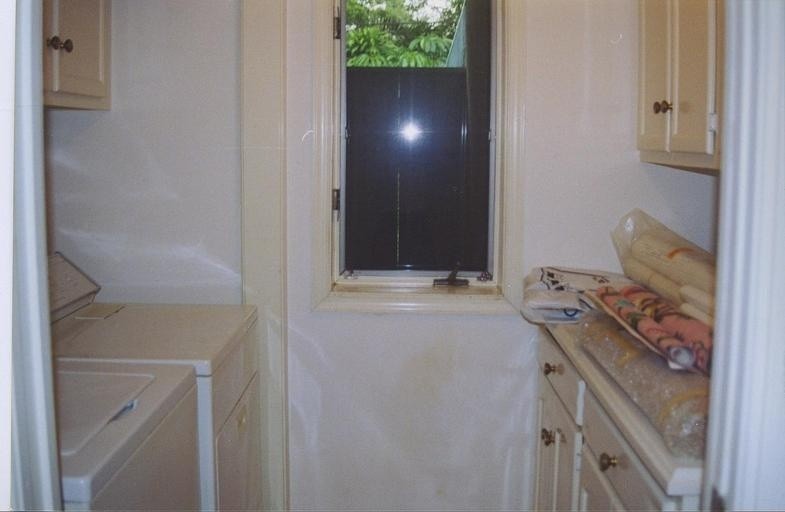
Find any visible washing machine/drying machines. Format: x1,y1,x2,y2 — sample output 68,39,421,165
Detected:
48,252,263,512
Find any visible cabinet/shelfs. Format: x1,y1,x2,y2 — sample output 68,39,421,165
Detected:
51,303,257,511
536,324,703,512
44,0,110,108
639,0,725,165
